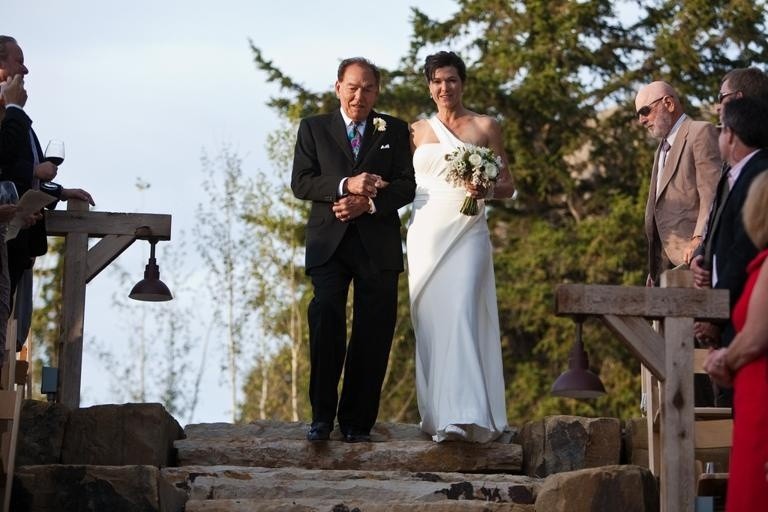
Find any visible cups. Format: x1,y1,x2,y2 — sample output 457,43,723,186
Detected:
703,462,722,475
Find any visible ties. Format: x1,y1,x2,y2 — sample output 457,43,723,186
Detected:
662,140,670,166
348,121,362,162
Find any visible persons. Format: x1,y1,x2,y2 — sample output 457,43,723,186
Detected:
689,98,768,408
375,51,515,443
702,168,767,511
715,66,767,120
635,80,723,286
291,55,417,442
0,35,96,376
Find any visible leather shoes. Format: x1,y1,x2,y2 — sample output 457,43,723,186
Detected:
346,434,370,442
308,422,332,440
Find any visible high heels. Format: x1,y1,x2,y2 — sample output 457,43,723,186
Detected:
445,424,468,441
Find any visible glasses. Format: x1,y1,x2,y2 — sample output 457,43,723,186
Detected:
717,91,736,104
636,95,674,120
715,123,725,132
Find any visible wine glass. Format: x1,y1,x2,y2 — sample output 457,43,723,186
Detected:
0,181,19,234
42,140,65,190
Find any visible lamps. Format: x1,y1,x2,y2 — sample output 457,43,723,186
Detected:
128,242,173,303
550,323,607,399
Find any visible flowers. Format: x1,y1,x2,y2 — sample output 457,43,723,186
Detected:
367,117,390,138
441,141,505,216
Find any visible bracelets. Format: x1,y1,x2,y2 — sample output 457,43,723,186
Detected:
485,189,495,200
